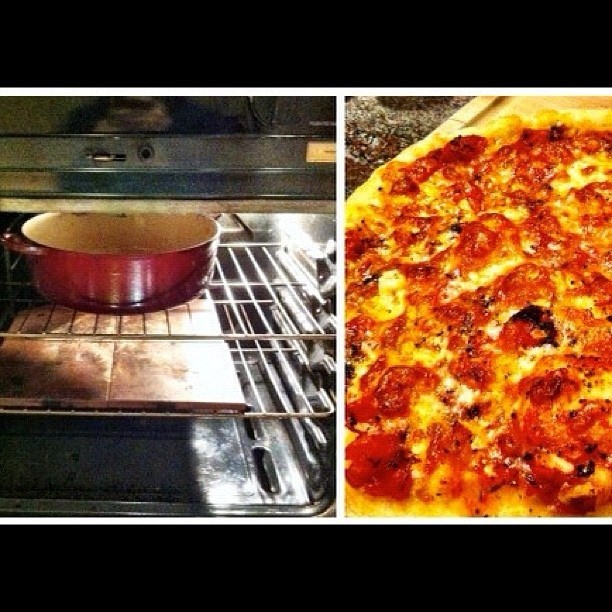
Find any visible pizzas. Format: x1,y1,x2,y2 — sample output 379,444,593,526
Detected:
342,106,611,519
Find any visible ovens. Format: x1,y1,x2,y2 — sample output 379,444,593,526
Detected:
1,97,336,517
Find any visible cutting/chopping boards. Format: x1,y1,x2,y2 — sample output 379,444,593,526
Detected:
0,304,250,412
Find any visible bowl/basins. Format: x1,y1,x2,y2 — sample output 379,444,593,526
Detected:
4,213,222,316
